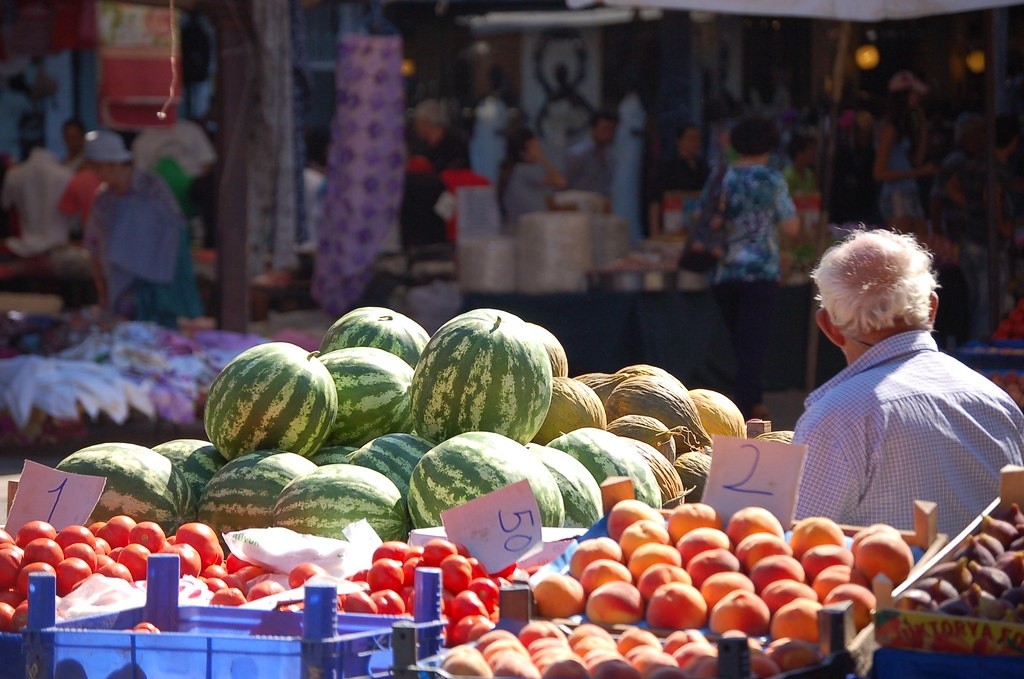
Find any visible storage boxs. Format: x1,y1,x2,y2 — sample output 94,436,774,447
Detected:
0,463,1024,679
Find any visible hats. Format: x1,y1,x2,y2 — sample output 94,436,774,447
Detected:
78,130,135,164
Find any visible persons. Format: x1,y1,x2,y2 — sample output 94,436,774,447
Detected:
790,232,1024,540
692,117,799,420
83,131,184,327
295,39,1024,349
0,118,216,318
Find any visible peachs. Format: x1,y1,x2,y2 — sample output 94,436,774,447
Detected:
445,496,913,678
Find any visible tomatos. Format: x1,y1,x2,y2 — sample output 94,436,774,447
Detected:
0,509,519,648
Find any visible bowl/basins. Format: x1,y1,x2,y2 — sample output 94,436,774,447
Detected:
602,265,677,293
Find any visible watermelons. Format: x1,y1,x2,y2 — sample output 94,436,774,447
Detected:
53,301,663,560
